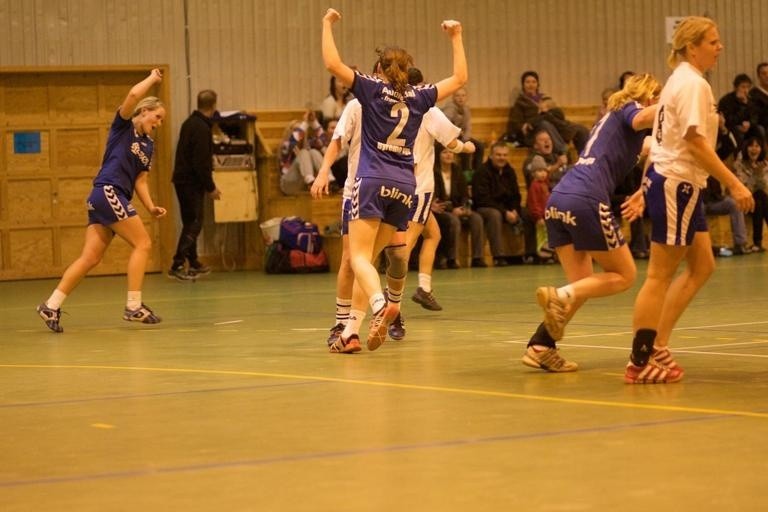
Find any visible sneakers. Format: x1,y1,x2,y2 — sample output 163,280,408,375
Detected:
650,344,685,374
168,267,192,283
306,180,341,194
411,286,442,312
623,353,684,387
388,310,406,340
367,303,398,351
519,345,579,373
35,302,64,333
188,265,212,279
326,323,346,346
122,301,162,324
535,285,573,342
328,333,362,354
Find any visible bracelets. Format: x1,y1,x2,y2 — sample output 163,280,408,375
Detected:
447,140,464,155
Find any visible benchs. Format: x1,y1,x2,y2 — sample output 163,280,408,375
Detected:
408,103,605,267
254,102,344,273
620,105,768,258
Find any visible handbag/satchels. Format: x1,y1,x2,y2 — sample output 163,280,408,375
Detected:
264,242,330,275
279,216,321,255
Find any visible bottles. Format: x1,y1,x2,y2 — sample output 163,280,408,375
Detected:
461,199,473,221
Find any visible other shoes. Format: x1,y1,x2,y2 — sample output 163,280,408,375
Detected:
748,245,761,253
471,257,489,268
498,258,508,266
522,255,543,265
541,258,554,264
631,248,651,258
446,259,461,269
733,245,747,253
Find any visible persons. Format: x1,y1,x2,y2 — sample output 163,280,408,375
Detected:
618,12,756,389
383,67,479,312
166,87,229,283
516,67,662,373
319,8,469,354
307,56,406,348
32,67,164,333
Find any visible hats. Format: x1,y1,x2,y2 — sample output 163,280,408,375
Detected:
530,155,548,173
